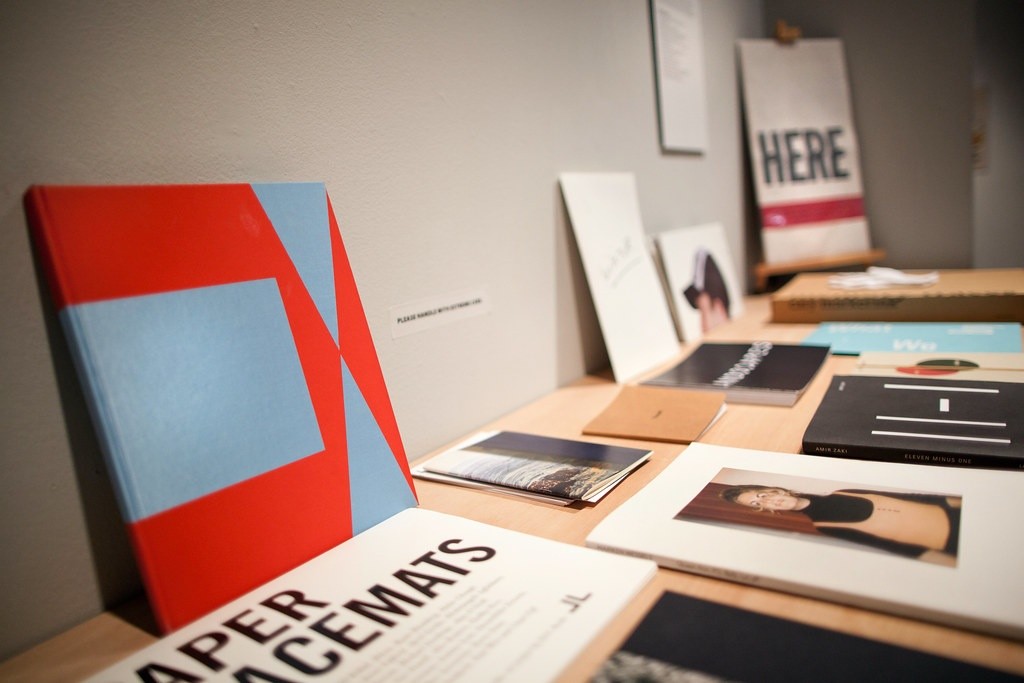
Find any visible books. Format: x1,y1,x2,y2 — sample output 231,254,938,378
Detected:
584,440,1023,632
81,501,1023,682
657,219,748,344
582,384,729,445
404,428,653,509
803,320,1023,373
801,374,1023,470
558,170,682,383
640,342,831,408
26,179,423,636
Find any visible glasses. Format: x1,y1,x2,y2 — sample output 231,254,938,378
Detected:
750,492,768,513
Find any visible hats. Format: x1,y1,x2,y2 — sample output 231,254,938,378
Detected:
683,250,731,318
